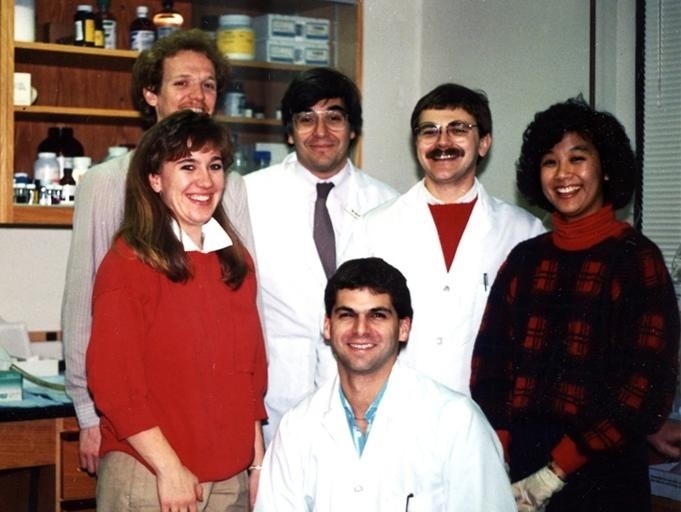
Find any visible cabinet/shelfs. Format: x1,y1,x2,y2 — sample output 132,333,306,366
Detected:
1,1,363,230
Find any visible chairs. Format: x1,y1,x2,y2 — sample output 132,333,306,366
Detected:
50,417,98,510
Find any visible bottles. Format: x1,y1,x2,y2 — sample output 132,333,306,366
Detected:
94,16,106,49
201,13,255,63
224,135,272,178
96,1,118,50
128,6,158,53
152,1,185,42
13,127,138,206
224,76,266,122
74,5,96,48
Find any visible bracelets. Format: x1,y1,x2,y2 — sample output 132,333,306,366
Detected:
546,463,570,486
245,462,267,472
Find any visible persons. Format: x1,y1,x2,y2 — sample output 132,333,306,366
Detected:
244,65,405,447
54,27,258,476
361,82,549,357
469,91,681,511
83,107,272,511
252,255,521,512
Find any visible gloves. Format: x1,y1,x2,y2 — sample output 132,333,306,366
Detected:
509,461,569,512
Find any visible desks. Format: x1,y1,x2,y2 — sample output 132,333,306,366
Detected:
0,348,104,512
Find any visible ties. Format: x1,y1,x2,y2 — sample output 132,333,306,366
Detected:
314,183,336,281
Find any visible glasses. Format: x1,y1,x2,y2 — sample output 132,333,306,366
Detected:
291,111,349,134
412,122,477,145
